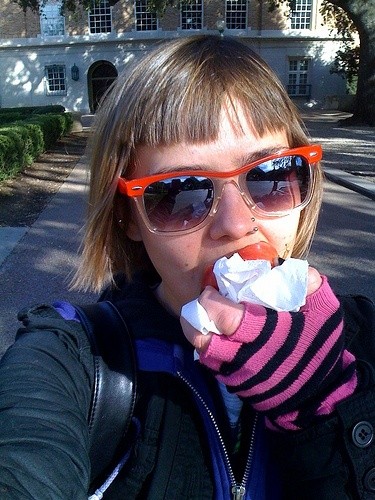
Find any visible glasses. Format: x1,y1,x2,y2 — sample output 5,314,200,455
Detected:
116,143,323,237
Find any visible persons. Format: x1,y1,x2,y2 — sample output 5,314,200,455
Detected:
147,183,183,232
1,34,374,500
